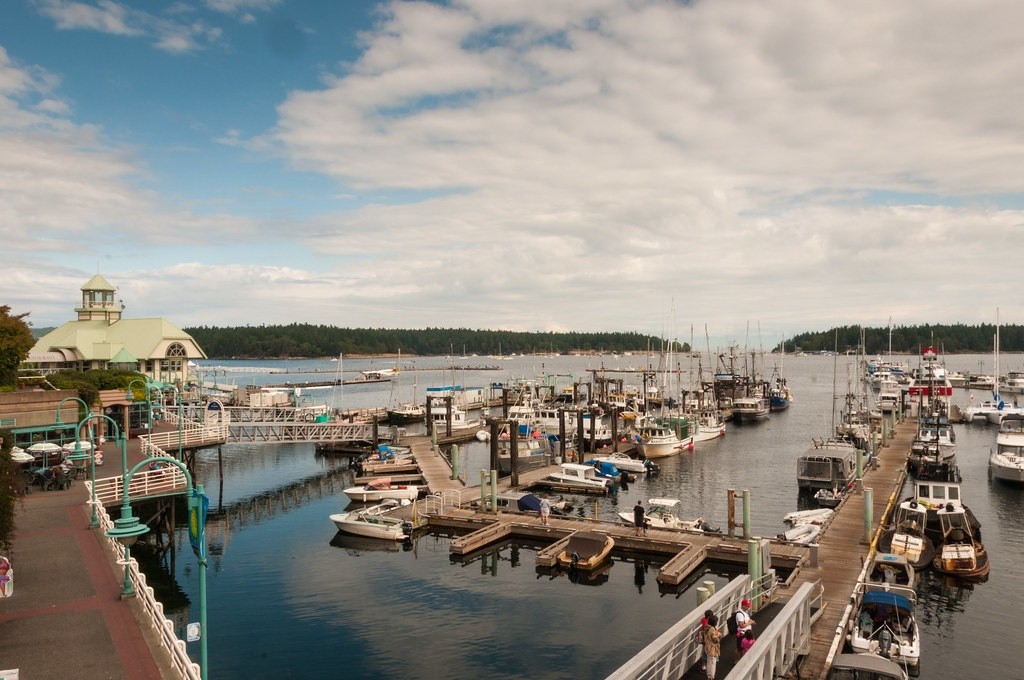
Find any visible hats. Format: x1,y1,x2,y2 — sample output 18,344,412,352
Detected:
741,599,752,607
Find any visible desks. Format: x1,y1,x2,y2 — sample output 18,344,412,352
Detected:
74,466,87,480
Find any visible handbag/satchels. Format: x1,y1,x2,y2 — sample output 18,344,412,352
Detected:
695,625,706,644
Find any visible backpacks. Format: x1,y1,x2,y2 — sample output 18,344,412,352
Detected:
727,611,745,635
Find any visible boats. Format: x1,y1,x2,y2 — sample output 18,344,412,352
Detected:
557,530,616,570
496,318,795,472
424,402,466,426
329,506,415,541
475,490,569,517
618,497,724,535
775,354,1024,680
584,451,662,483
343,476,420,502
387,404,426,424
537,462,616,495
355,446,414,468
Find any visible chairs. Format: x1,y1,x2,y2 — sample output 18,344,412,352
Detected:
22,463,92,493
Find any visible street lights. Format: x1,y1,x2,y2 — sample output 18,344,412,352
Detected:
184,373,218,422
152,384,183,463
52,396,99,525
65,413,136,595
124,379,152,459
103,455,209,679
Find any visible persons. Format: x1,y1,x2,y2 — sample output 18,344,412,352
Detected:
734,600,756,665
634,499,644,536
635,564,645,593
539,494,550,526
390,426,400,445
154,410,160,426
60,459,70,476
699,610,722,680
1013,396,1018,408
